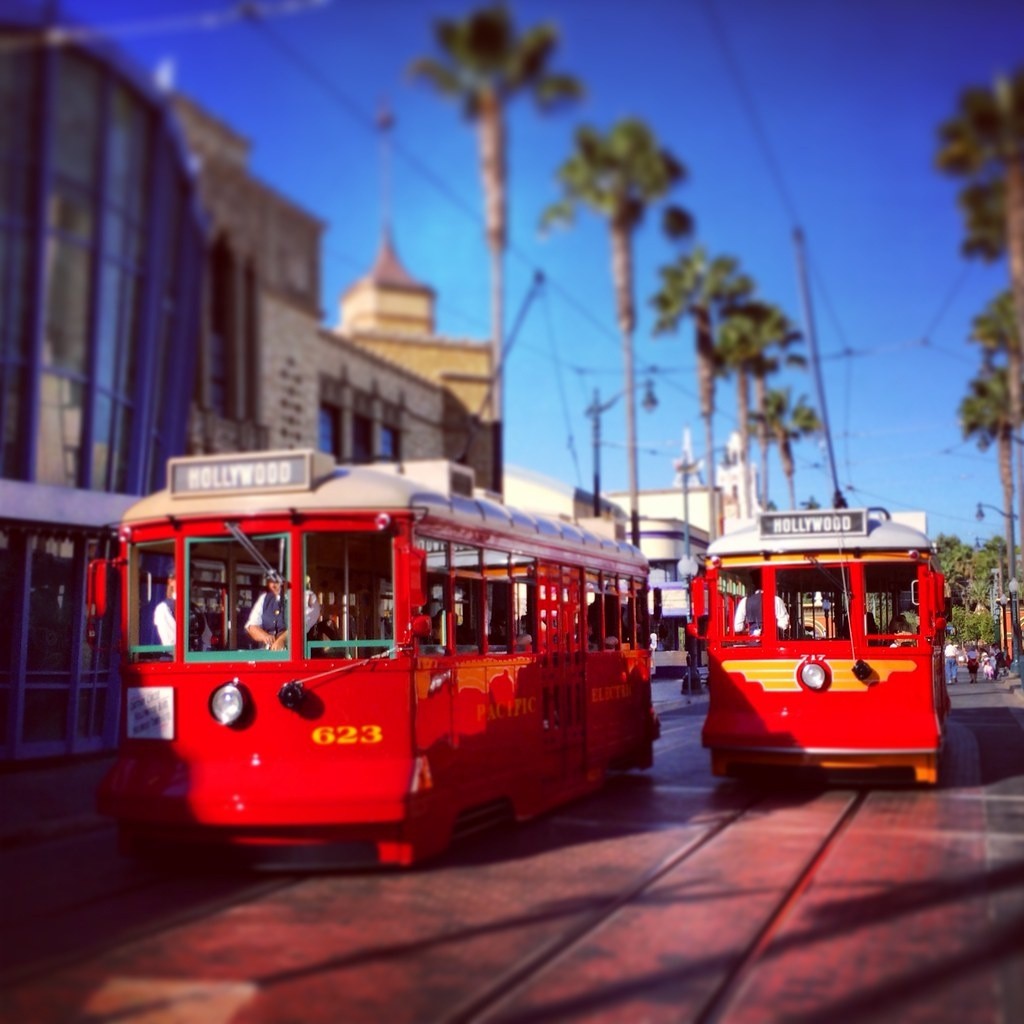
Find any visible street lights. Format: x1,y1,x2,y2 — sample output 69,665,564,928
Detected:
675,552,706,695
584,376,660,515
977,502,1017,576
1007,577,1022,674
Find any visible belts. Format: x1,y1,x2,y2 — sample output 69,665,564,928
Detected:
949,657,956,658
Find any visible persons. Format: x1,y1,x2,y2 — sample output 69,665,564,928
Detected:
944,640,1012,685
153,561,394,658
734,569,789,645
421,593,644,646
841,611,920,646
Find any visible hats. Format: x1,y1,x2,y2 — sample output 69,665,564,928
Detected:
167,561,195,577
262,566,279,586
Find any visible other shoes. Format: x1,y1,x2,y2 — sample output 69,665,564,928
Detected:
970,679,974,683
954,677,957,682
975,681,977,684
947,683,952,685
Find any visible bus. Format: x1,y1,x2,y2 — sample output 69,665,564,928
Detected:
88,451,660,870
687,508,957,793
